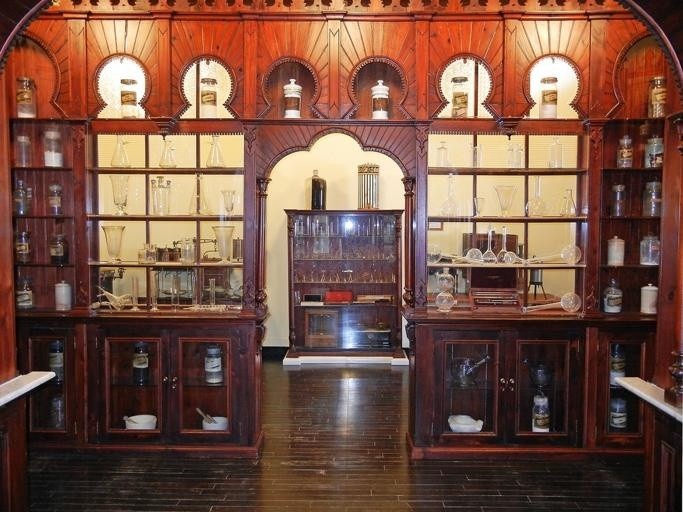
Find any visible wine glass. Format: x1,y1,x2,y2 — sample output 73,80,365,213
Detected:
450,358,479,389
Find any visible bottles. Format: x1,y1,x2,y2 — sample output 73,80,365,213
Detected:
603,277,659,315
199,77,219,118
48,341,64,381
437,172,577,218
614,134,663,168
14,131,72,314
282,78,303,118
437,134,562,169
532,360,546,384
647,76,668,119
609,344,627,387
295,216,398,283
450,76,469,119
606,232,661,265
311,170,325,211
539,76,559,118
133,344,149,385
204,347,222,384
119,77,140,120
46,395,65,431
370,79,390,119
429,223,582,267
611,393,630,431
531,395,550,433
101,133,234,312
607,178,662,218
428,268,581,314
16,77,37,119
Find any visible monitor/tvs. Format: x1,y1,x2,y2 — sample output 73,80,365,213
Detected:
468,265,519,292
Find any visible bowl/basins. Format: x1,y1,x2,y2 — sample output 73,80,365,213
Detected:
448,414,482,432
201,416,229,430
122,414,157,431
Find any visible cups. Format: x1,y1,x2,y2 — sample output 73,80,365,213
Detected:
391,296,395,305
